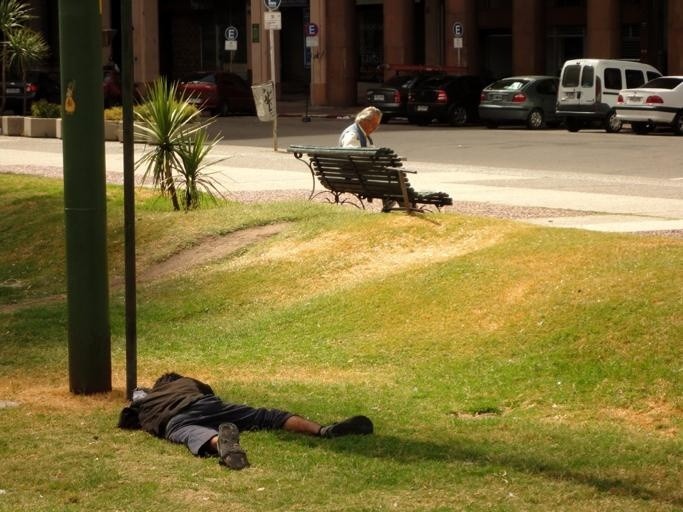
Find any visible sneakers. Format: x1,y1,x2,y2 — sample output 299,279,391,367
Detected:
217,422,250,470
319,416,373,438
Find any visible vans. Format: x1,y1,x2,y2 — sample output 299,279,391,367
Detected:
554,59,663,132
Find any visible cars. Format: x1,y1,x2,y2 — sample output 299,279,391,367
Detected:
478,75,560,129
102,65,121,102
174,72,252,117
1,70,59,113
366,71,446,120
615,76,682,136
404,73,504,127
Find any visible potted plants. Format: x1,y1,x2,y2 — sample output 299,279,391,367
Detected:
22,99,203,146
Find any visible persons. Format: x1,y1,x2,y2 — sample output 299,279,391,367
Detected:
338,106,416,212
119,373,372,470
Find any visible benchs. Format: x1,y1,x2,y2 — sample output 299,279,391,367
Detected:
285,143,453,216
